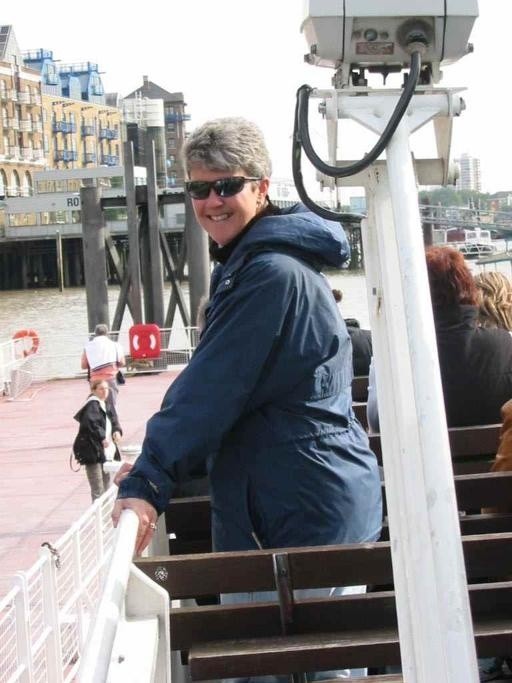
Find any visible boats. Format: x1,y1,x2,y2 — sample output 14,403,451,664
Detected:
473,238,512,266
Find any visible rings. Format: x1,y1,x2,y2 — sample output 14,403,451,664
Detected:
141,514,149,525
154,526,157,529
149,522,156,528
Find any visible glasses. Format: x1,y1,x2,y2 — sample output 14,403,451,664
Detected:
184,175,263,200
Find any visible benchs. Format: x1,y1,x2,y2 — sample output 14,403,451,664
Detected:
367,423,512,541
170,471,511,606
134,532,512,682
351,376,370,431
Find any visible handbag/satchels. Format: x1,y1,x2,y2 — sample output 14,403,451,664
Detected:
74,433,102,465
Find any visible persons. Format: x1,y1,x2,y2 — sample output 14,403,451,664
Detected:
72,378,123,499
367,242,512,434
336,198,341,208
481,399,512,586
112,116,386,682
472,270,512,331
79,322,126,404
344,315,372,375
331,287,342,304
